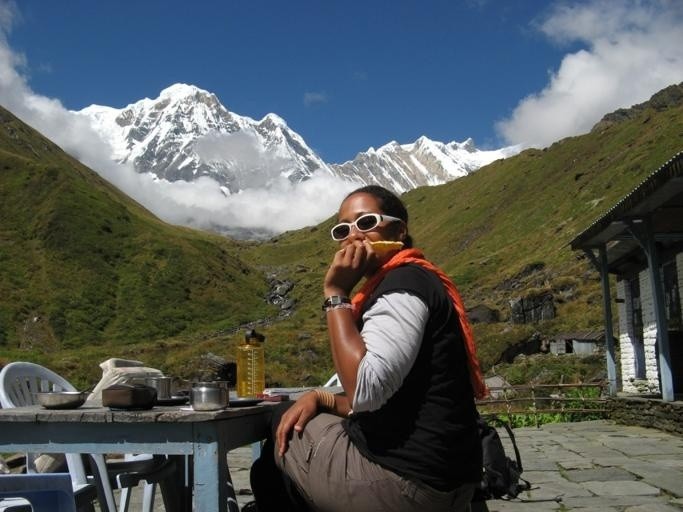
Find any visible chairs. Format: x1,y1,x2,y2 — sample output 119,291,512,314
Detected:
0,360,177,512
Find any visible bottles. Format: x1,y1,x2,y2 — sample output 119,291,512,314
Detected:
236,328,265,399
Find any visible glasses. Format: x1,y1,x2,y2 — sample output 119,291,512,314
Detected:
330,213,400,241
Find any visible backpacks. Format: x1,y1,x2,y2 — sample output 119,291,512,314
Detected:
479,417,530,497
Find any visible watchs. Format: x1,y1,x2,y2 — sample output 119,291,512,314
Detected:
320,294,351,311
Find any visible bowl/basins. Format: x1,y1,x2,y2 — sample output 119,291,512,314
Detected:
30,390,90,411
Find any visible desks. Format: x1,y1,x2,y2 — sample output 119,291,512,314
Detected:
0,386,346,512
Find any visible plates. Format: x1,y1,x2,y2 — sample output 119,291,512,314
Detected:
229,398,266,407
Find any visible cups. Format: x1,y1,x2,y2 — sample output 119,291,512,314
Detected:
145,375,172,401
189,380,231,412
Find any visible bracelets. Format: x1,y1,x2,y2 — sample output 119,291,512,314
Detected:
312,388,335,412
325,302,356,312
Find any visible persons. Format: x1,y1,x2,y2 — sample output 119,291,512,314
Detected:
249,186,489,511
